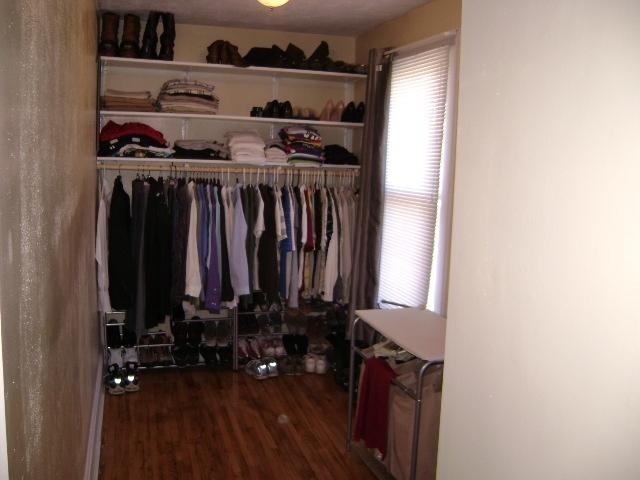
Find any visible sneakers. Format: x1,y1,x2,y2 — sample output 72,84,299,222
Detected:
171,287,350,383
107,348,123,370
139,333,155,367
154,330,172,366
103,364,124,396
107,318,121,347
123,360,141,393
122,317,138,348
124,347,138,370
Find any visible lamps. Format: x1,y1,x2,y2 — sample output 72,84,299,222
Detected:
256,1,289,9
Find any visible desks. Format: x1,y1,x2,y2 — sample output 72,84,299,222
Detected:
344,308,444,480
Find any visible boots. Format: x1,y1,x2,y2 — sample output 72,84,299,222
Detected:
119,12,142,58
157,10,177,61
140,7,159,61
98,11,122,59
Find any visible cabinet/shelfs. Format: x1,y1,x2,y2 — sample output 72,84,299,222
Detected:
95,55,371,370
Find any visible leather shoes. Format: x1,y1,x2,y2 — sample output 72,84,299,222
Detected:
204,38,252,69
261,95,366,124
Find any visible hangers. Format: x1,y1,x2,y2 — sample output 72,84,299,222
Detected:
96,162,358,199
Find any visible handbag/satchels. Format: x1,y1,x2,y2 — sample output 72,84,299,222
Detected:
352,347,444,480
